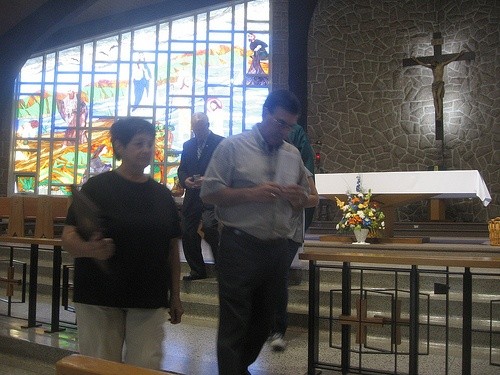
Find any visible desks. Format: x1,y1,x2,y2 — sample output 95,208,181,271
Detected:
315,169,492,221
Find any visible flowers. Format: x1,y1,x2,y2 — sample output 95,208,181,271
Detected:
333,175,386,232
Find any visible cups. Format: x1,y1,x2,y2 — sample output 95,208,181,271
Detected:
193,173,201,187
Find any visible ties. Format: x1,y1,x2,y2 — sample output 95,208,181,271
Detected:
197,143,203,159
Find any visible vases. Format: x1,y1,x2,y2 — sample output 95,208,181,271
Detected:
352,229,370,245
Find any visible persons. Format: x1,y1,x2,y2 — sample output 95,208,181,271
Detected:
198,89,320,375
265,124,320,349
62,116,184,371
177,113,225,281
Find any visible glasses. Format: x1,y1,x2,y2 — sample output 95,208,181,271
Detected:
273,117,296,134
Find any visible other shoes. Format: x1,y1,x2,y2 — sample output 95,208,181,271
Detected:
270,332,287,351
183,274,207,280
